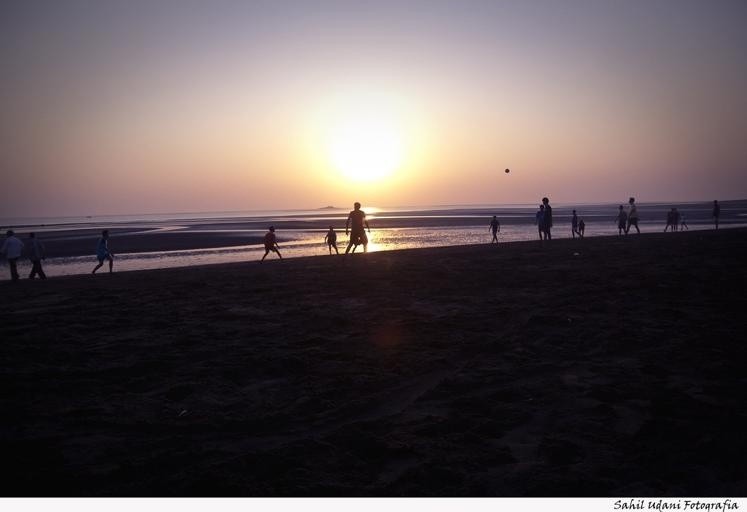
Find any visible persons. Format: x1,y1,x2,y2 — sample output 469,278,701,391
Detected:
324,225,339,255
344,202,371,255
0,230,25,281
487,195,722,243
91,227,115,274
258,224,285,264
23,231,48,279
352,236,368,255
